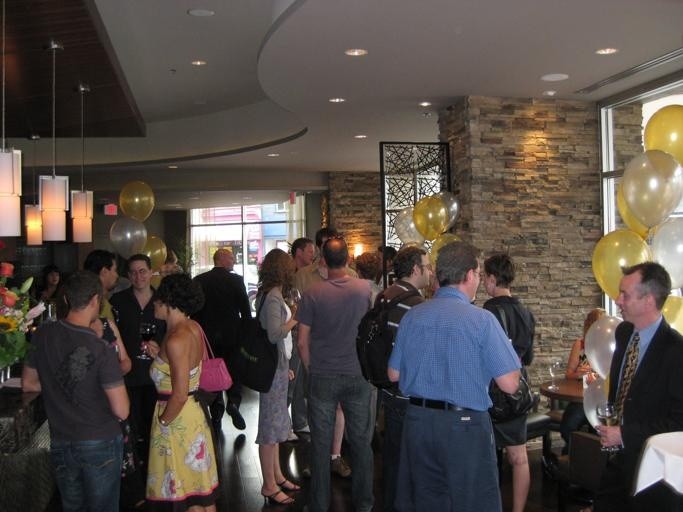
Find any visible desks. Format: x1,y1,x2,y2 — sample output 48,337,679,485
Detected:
540,379,583,412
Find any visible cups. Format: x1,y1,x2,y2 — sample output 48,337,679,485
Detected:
287,287,301,304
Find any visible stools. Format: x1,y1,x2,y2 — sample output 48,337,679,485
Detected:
496,412,551,492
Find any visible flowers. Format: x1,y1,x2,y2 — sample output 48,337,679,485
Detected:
0,263,46,368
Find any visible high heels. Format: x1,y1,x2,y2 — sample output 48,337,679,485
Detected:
277,478,302,492
261,488,294,507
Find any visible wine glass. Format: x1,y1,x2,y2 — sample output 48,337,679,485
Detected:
594,401,623,453
135,320,160,361
546,358,561,392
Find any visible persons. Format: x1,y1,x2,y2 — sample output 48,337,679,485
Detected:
256,228,381,511
387,241,523,511
594,262,683,512
140,273,219,511
484,254,535,511
560,307,606,457
23,249,132,511
109,255,167,511
191,249,252,435
371,246,430,510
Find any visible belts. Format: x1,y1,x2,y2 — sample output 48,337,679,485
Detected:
409,396,473,412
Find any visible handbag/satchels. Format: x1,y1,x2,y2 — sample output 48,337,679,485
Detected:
201,358,232,392
231,317,278,392
490,374,534,422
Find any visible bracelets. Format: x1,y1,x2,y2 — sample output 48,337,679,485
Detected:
159,417,168,426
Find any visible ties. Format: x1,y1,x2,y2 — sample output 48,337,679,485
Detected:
615,332,640,425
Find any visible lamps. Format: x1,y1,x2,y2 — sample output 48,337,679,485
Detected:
38,43,69,242
352,244,364,261
103,203,118,216
72,85,93,243
0,1,22,237
24,136,42,245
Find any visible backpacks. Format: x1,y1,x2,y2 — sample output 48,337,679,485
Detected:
356,291,420,389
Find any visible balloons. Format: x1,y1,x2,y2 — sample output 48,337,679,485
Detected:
393,190,463,272
109,180,167,274
582,104,683,433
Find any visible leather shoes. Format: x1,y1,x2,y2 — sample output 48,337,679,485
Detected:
226,401,246,429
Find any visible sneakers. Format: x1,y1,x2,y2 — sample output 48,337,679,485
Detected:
286,429,297,441
330,456,353,478
